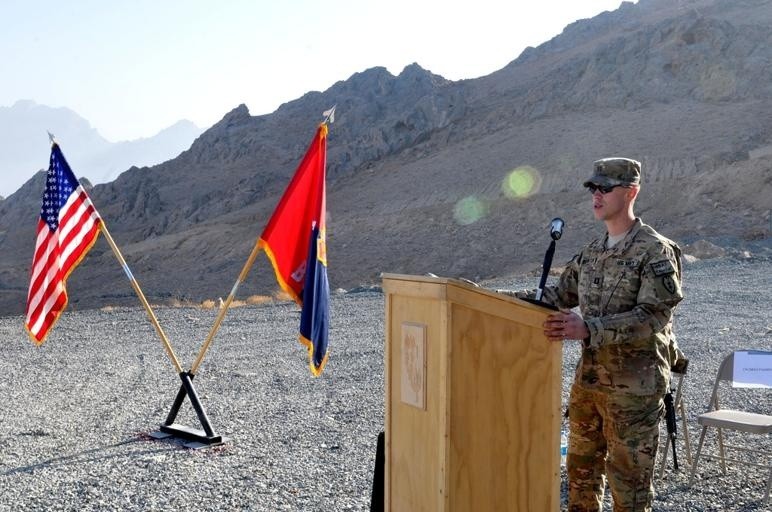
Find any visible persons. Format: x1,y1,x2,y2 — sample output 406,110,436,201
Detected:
490,157,684,510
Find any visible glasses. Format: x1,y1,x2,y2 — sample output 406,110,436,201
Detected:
589,183,621,194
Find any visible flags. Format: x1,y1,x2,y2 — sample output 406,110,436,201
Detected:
257,121,330,380
22,142,104,347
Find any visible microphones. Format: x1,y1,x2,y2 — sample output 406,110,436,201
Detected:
550,218,564,243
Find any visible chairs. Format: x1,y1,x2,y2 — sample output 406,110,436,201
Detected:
692,347,771,489
621,353,695,479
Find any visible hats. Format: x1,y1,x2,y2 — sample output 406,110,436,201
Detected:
583,157,641,188
